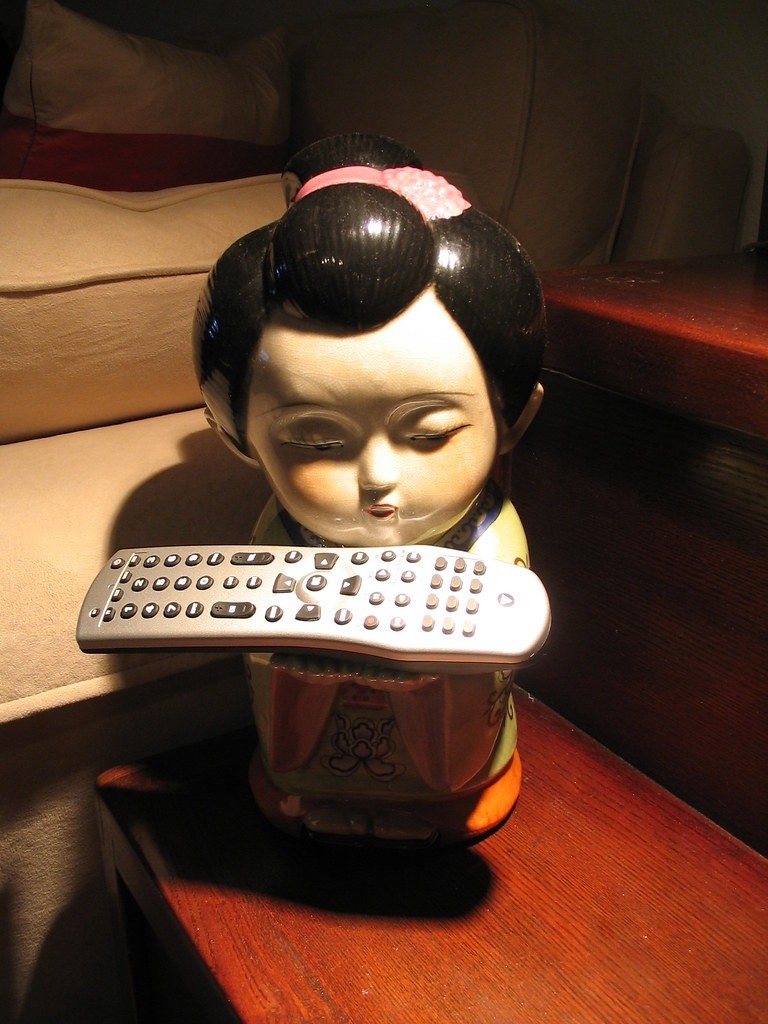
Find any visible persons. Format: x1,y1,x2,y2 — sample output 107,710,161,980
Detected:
193,133,549,843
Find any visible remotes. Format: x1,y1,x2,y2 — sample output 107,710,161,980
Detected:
75,545,553,680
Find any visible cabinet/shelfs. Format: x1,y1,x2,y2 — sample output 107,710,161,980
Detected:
512,252,768,851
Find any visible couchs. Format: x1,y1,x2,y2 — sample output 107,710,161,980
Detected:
0,0,752,752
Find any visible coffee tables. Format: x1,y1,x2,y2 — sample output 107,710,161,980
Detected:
94,685,768,1024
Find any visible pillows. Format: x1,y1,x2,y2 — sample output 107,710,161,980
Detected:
3,0,294,155
